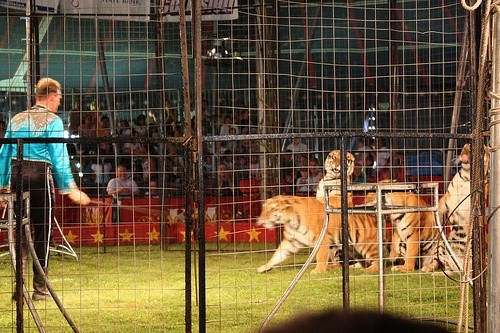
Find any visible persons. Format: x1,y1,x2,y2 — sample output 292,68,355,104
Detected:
69,97,405,198
0,77,90,303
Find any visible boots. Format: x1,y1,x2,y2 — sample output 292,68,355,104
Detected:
32,267,54,301
